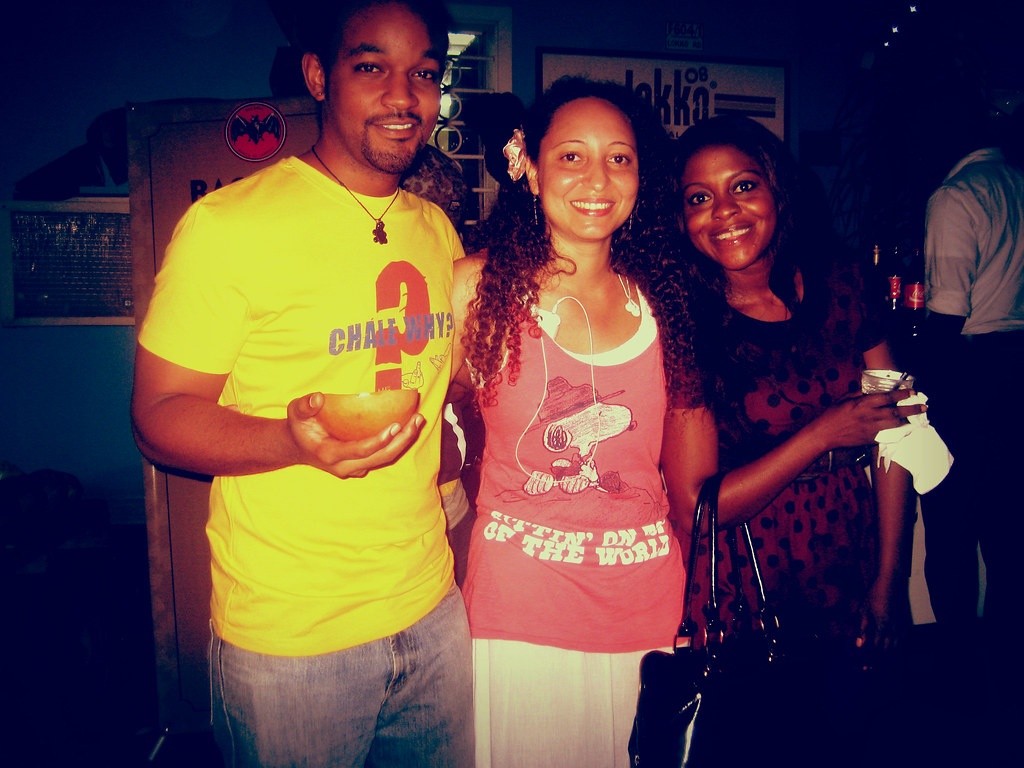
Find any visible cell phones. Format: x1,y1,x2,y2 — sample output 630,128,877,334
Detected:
530,306,560,341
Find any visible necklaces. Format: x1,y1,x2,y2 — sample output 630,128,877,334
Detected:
312,145,399,243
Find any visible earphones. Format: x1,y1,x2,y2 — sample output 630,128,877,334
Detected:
632,303,641,317
625,299,635,312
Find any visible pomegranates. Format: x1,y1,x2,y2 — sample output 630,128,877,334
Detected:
312,388,417,441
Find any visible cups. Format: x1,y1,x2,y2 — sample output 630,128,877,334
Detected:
861,369,915,393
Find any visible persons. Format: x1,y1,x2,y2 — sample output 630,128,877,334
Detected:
644,115,907,650
908,92,1024,660
440,77,688,768
130,0,475,768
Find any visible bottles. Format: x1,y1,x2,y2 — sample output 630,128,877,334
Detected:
875,246,925,345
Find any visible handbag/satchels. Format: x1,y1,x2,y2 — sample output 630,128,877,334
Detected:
628,471,814,768
